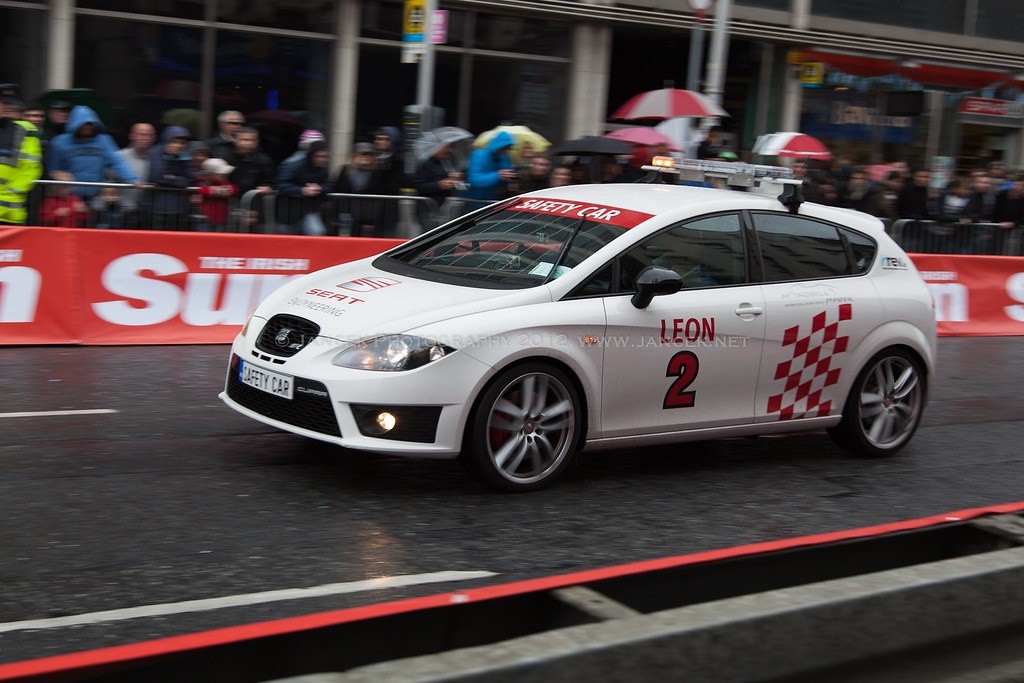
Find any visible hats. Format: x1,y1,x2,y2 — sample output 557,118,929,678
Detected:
0,83,29,105
297,129,327,145
367,127,403,146
199,157,237,175
354,142,377,154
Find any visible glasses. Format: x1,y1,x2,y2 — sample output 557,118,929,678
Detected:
219,121,247,127
375,136,392,142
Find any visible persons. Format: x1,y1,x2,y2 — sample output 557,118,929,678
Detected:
465,132,520,212
196,159,239,236
113,122,156,227
27,87,94,225
0,84,43,225
209,112,246,157
648,120,1024,239
405,142,465,232
520,153,555,194
187,139,210,167
335,126,403,237
549,165,571,188
274,140,330,236
47,106,144,226
225,130,268,199
144,126,199,229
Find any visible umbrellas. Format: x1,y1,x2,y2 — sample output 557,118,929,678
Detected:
611,87,732,119
413,127,473,190
549,136,633,155
753,132,832,161
604,128,684,152
474,124,551,156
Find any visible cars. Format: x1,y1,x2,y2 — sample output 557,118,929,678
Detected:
217,183,937,492
